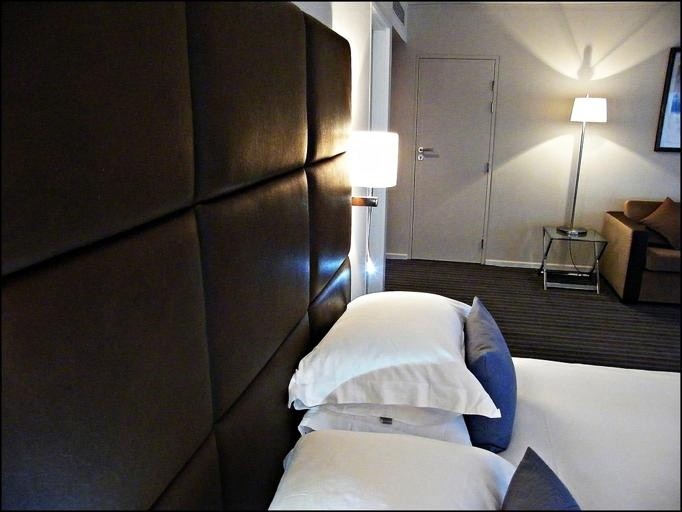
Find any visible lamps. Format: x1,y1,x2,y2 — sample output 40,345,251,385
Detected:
556,97,609,237
352,129,401,296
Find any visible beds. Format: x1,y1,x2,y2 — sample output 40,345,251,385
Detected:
473,357,680,512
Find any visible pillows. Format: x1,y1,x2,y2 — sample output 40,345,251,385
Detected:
298,409,472,446
638,197,681,250
463,296,518,452
268,430,516,511
502,446,582,511
288,290,502,424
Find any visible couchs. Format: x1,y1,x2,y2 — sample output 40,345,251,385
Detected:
596,200,680,307
